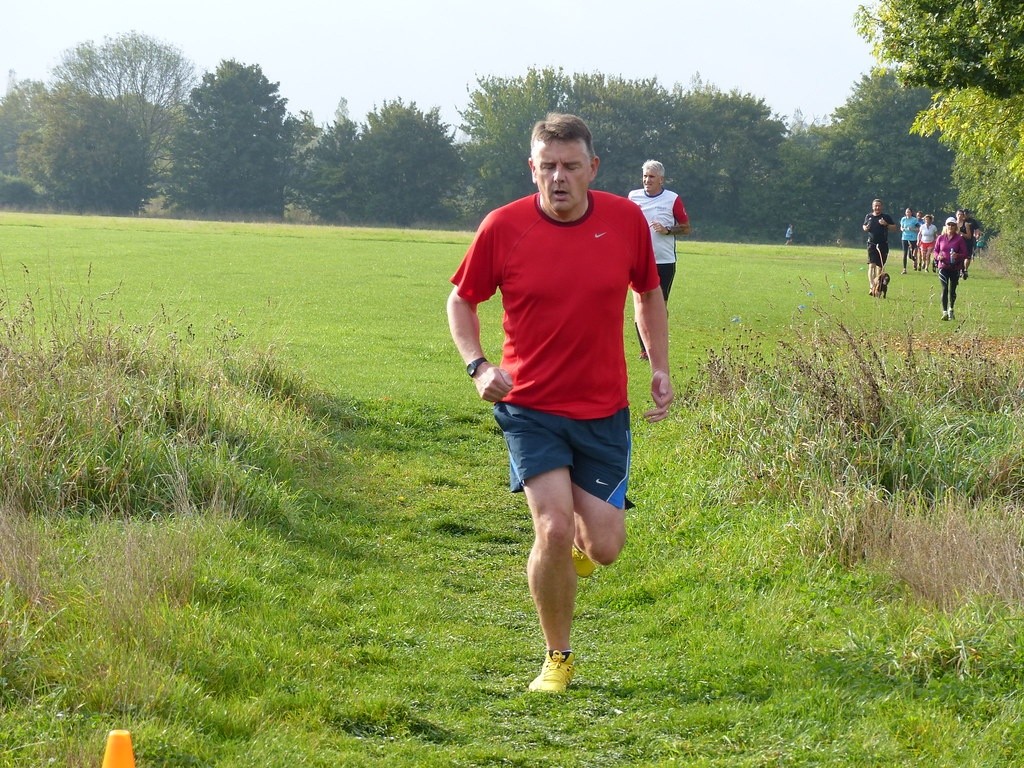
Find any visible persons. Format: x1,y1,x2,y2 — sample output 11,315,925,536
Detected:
862,199,897,297
446,112,673,693
900,208,919,274
909,211,937,273
785,224,794,245
934,217,967,321
628,159,691,360
956,209,989,280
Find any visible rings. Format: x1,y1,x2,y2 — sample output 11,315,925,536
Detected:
666,409,670,413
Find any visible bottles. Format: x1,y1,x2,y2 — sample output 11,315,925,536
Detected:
868,217,872,229
950,248,954,263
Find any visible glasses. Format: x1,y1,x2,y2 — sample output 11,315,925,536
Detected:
947,223,956,226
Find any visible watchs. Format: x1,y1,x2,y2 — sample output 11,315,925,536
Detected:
466,358,488,378
665,226,671,235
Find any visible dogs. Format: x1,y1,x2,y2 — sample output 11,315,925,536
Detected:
873,273,890,299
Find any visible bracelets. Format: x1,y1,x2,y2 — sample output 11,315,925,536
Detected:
884,222,889,227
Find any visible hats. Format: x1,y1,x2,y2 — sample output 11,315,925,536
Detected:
945,217,957,226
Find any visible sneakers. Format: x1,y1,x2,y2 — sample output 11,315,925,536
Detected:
572,544,595,579
529,651,575,694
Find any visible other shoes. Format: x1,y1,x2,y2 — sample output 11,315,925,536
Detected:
941,311,948,321
960,272,962,277
921,263,924,268
964,271,968,277
913,261,917,271
901,271,906,274
949,308,955,321
925,268,929,273
869,288,873,296
919,264,921,271
